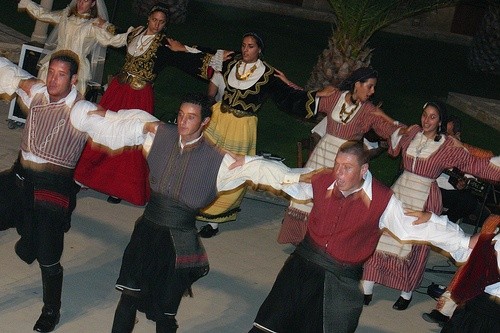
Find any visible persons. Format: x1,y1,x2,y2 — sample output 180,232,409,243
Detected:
88,93,333,333
0,57,45,97
220,139,497,333
165,31,341,239
92,4,234,204
272,67,418,254
0,49,164,332
18,0,133,98
403,207,500,333
434,117,496,224
362,101,500,311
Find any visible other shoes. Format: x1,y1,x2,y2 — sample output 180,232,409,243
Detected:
392,296,412,310
363,295,372,305
423,310,449,327
107,195,121,204
198,224,218,238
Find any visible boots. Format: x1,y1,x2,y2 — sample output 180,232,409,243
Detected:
156,320,178,333
33,266,63,333
111,293,139,333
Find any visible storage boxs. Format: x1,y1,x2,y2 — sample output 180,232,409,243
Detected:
8,41,58,124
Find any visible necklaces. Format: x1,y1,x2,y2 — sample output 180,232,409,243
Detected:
235,61,256,80
340,104,360,123
140,36,156,51
416,131,437,157
